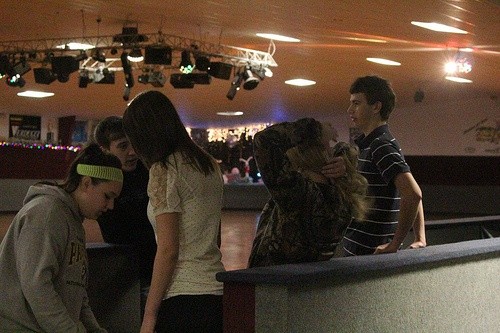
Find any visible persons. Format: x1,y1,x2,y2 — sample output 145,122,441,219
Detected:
342,76,427,258
122,90,226,333
0,138,124,333
94,116,157,323
246,117,369,268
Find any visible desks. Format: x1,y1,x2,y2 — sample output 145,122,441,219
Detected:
409,215,500,239
222,183,270,209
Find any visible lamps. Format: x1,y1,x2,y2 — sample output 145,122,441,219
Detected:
0,18,278,101
445,48,474,84
